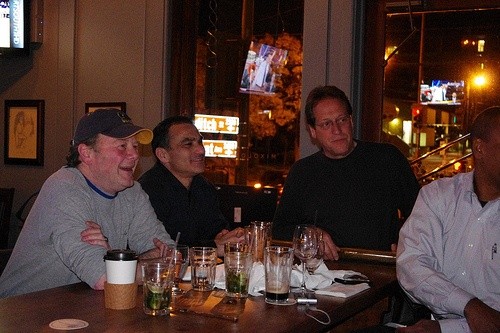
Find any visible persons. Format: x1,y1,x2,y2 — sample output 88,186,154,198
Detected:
273,86,422,260
396,106,500,333
137,115,251,256
0,107,176,300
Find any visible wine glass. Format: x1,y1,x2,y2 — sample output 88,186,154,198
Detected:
161,243,190,297
292,224,320,300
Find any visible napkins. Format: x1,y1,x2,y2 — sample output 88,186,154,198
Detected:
315,282,370,298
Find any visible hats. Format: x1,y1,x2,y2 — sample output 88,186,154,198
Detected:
74,108,154,145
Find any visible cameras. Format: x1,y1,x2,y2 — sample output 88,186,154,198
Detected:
294,292,317,305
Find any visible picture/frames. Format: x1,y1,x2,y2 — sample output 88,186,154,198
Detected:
3,98,45,167
85,102,126,114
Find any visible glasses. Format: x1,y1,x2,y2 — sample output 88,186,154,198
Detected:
314,113,349,131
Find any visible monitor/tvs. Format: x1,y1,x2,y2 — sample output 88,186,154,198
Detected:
0,0,30,55
237,38,289,96
421,78,465,106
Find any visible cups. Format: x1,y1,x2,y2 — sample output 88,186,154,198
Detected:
223,242,253,298
249,220,273,265
141,257,176,317
305,240,325,276
263,246,294,303
102,248,139,285
189,246,217,292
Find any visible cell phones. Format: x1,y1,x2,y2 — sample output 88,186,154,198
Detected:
334,277,371,284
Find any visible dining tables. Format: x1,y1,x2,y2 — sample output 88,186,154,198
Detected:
0,240,399,333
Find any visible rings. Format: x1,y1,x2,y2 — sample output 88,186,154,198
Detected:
103,235,108,242
